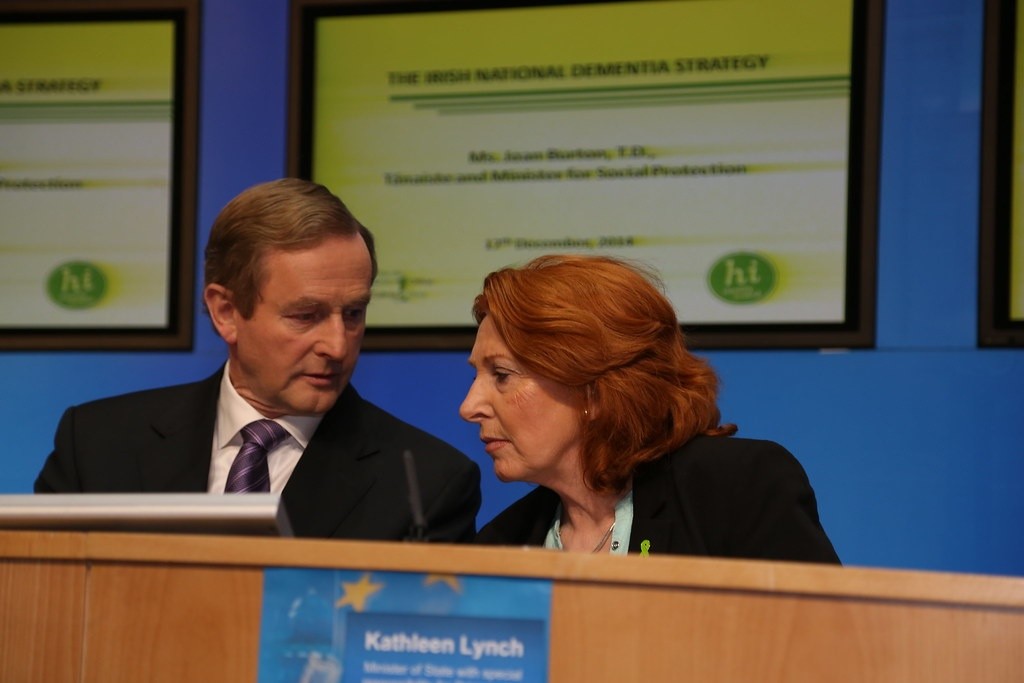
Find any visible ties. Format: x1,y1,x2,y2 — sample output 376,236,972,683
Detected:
224,419,292,493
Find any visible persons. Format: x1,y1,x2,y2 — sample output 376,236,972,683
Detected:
32,177,480,546
460,254,843,566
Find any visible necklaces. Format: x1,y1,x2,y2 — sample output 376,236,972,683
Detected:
592,522,616,553
